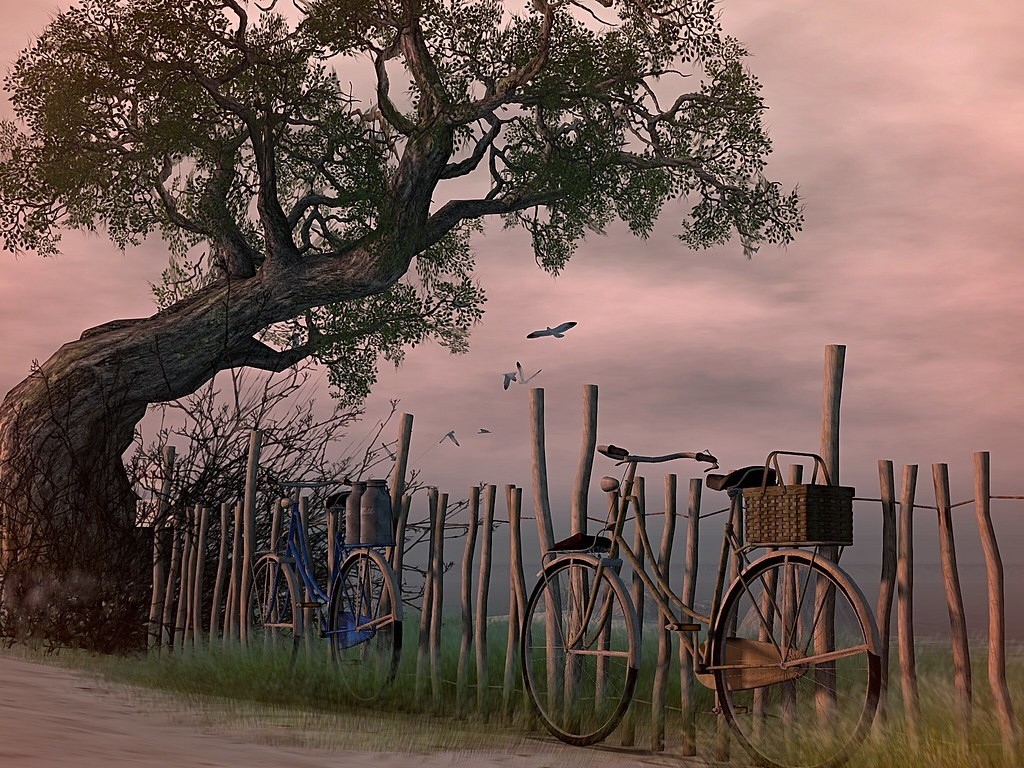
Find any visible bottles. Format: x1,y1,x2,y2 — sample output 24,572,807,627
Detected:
360,479,392,544
345,480,366,544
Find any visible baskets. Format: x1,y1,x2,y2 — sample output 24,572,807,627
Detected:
742,451,856,546
